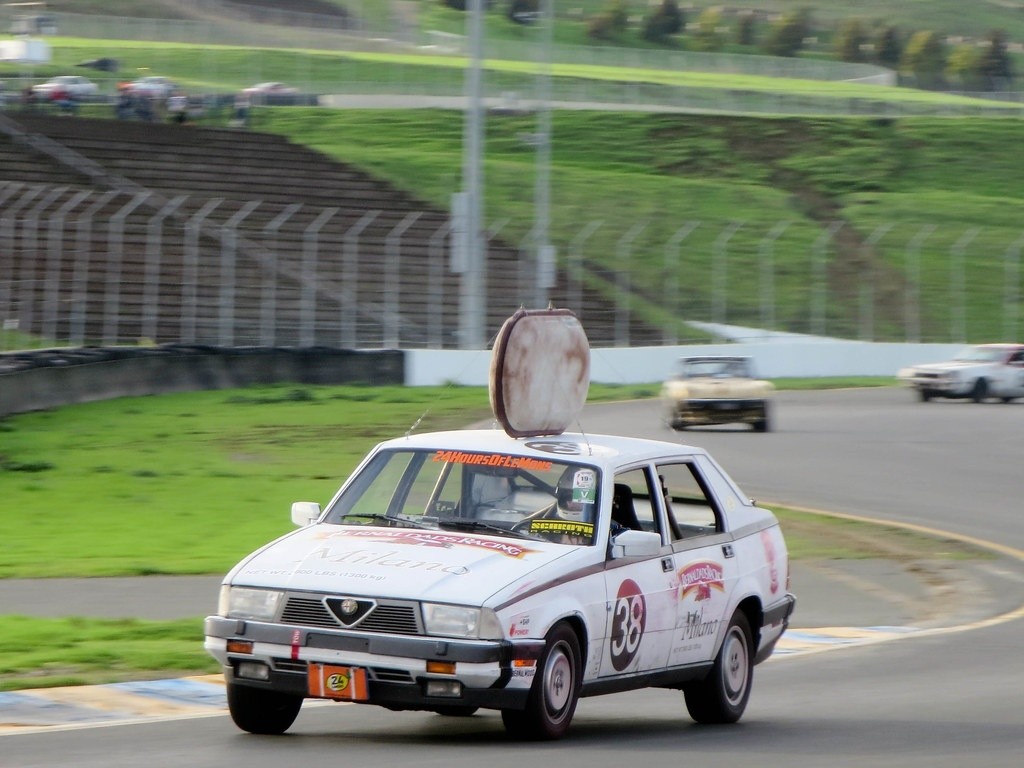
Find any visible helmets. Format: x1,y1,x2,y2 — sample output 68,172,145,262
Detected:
556,465,599,523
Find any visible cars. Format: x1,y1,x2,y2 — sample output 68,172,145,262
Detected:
28,75,98,103
203,430,796,743
240,81,321,108
898,344,1024,404
121,74,192,106
660,354,776,431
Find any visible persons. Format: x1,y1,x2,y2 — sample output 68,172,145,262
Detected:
517,467,632,544
